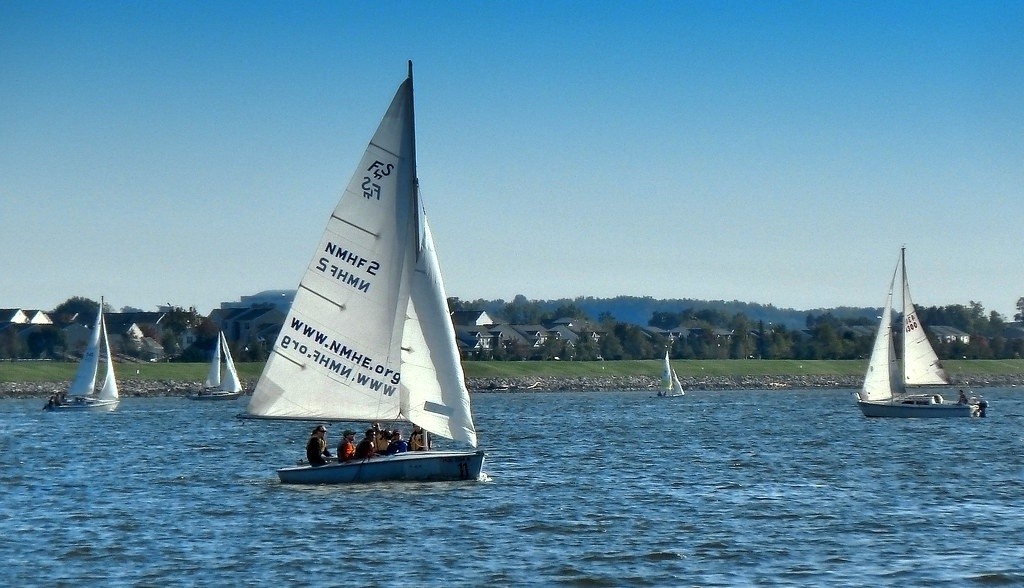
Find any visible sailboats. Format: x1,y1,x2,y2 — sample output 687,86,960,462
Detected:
651,350,686,399
244,59,488,487
186,330,245,401
850,245,990,418
42,294,120,414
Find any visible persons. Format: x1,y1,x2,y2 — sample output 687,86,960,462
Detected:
307,425,333,467
657,390,667,396
49,394,66,406
953,389,968,406
336,430,356,463
354,422,407,458
198,390,203,396
407,423,432,451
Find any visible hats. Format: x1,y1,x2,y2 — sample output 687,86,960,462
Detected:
343,430,356,436
392,429,401,435
313,425,326,435
